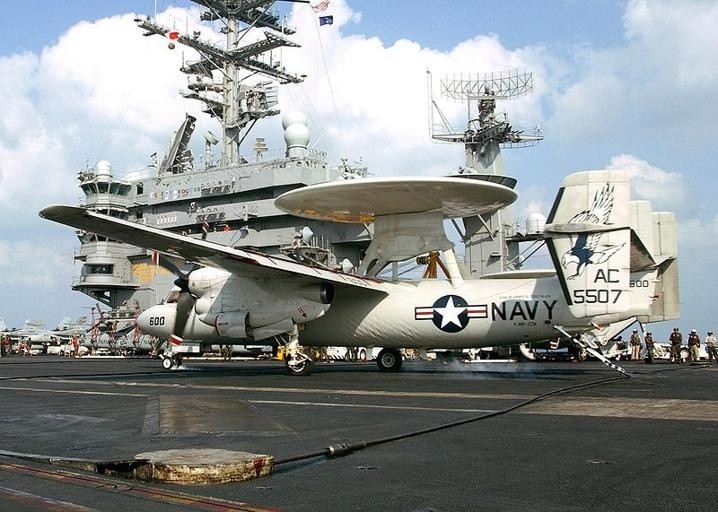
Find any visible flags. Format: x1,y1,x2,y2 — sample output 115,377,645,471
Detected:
319,15,334,26
312,0,331,13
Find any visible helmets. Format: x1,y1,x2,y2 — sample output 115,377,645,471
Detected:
647,331,652,335
707,329,713,335
674,328,679,332
633,329,637,333
691,329,697,334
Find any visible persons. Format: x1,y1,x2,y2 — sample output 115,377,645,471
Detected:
630,329,640,361
686,329,701,360
645,331,656,361
669,328,683,364
705,330,718,363
